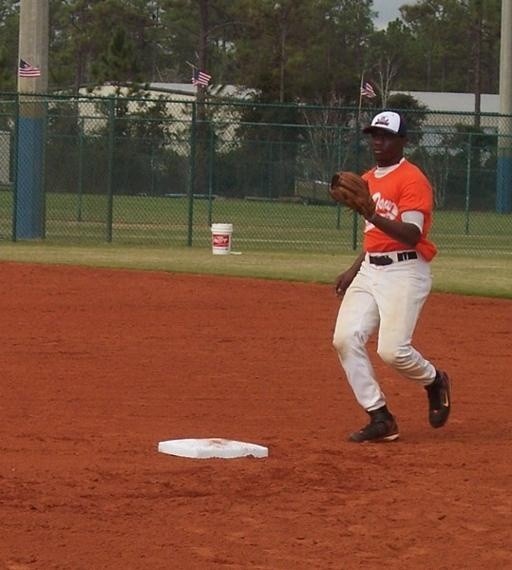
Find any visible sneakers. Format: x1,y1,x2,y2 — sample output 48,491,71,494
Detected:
424,370,450,428
350,416,399,442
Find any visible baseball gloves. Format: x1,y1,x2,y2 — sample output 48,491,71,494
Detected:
329,172,375,217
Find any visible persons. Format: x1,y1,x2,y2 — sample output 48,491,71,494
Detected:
330,112,449,443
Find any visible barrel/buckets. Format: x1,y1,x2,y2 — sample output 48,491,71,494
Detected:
210,223,234,256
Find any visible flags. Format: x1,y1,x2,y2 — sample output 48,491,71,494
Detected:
19,56,41,77
193,67,212,85
361,81,376,98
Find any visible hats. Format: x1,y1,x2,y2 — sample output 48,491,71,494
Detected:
363,109,406,137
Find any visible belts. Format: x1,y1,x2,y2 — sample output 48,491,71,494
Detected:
369,251,417,265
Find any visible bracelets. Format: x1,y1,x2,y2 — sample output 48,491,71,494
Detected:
369,212,378,220
